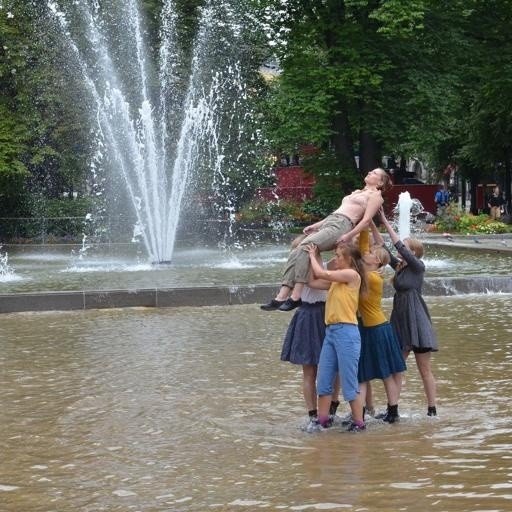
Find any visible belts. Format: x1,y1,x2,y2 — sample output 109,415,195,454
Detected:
331,212,355,230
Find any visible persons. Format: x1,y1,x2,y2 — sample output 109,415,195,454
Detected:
432,184,511,221
279,204,441,433
258,166,395,313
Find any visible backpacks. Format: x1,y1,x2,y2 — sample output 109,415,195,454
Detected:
434,191,444,203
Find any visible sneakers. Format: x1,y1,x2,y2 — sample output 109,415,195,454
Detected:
260,298,286,312
300,415,399,434
279,298,303,311
